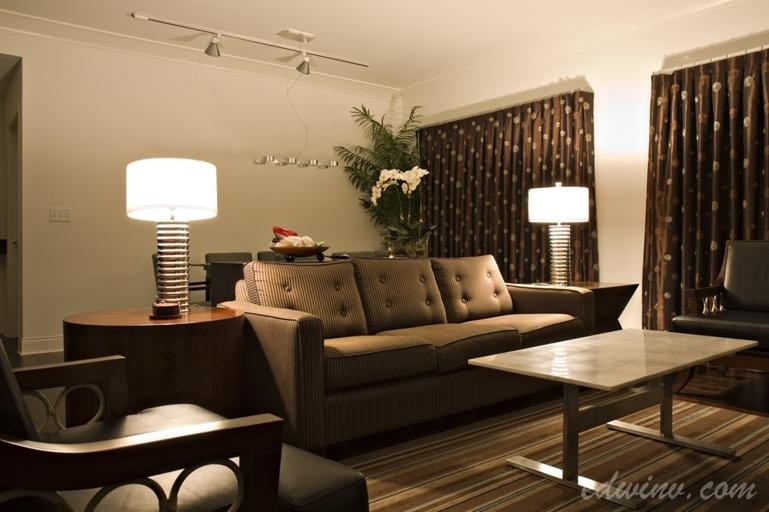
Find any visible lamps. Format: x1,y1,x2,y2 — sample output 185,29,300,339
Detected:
125,157,218,313
526,186,589,284
206,33,222,57
294,52,316,74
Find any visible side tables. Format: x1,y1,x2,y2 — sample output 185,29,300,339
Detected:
542,278,639,333
62,305,245,423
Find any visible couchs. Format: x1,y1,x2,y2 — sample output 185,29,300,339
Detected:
215,257,598,453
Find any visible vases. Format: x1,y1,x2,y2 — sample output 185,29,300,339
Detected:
385,224,434,258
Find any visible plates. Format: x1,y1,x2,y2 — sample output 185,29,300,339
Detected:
268,244,329,258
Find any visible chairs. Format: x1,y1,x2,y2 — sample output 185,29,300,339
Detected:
332,251,373,259
152,252,207,305
0,337,368,511
374,250,389,256
257,252,317,261
671,240,768,391
205,252,253,302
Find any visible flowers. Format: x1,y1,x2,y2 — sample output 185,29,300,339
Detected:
336,104,430,229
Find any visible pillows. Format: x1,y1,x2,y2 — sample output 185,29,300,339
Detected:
243,254,512,337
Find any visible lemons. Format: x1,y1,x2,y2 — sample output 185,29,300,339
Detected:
275,240,294,247
